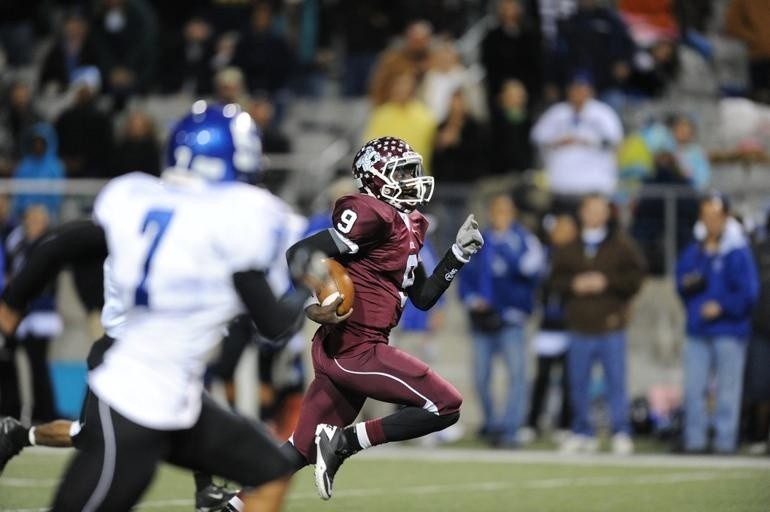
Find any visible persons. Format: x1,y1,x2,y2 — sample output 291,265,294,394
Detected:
201,132,484,511
356,1,760,451
0,2,310,428
46,99,322,511
0,410,241,511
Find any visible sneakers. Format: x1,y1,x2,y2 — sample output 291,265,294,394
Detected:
195,485,241,512
314,423,345,501
0,416,22,476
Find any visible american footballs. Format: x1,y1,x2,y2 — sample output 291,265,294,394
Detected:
306,252,354,317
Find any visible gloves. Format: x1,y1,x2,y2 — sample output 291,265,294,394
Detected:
455,213,485,256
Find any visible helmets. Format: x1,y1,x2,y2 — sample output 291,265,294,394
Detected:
350,136,435,213
164,102,265,184
